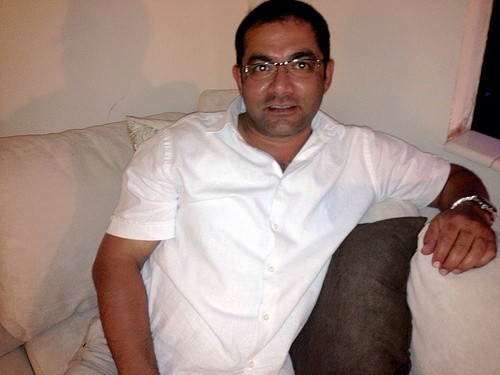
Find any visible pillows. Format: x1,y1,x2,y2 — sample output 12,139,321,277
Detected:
287,215,428,375
125,115,175,152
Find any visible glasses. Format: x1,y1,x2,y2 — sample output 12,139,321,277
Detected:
241,59,325,81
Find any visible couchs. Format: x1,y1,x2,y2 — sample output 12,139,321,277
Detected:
0,88,500,375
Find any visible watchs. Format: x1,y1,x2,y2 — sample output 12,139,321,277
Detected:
451,194,497,226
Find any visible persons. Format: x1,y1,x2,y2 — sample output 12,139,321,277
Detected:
62,0,497,375
